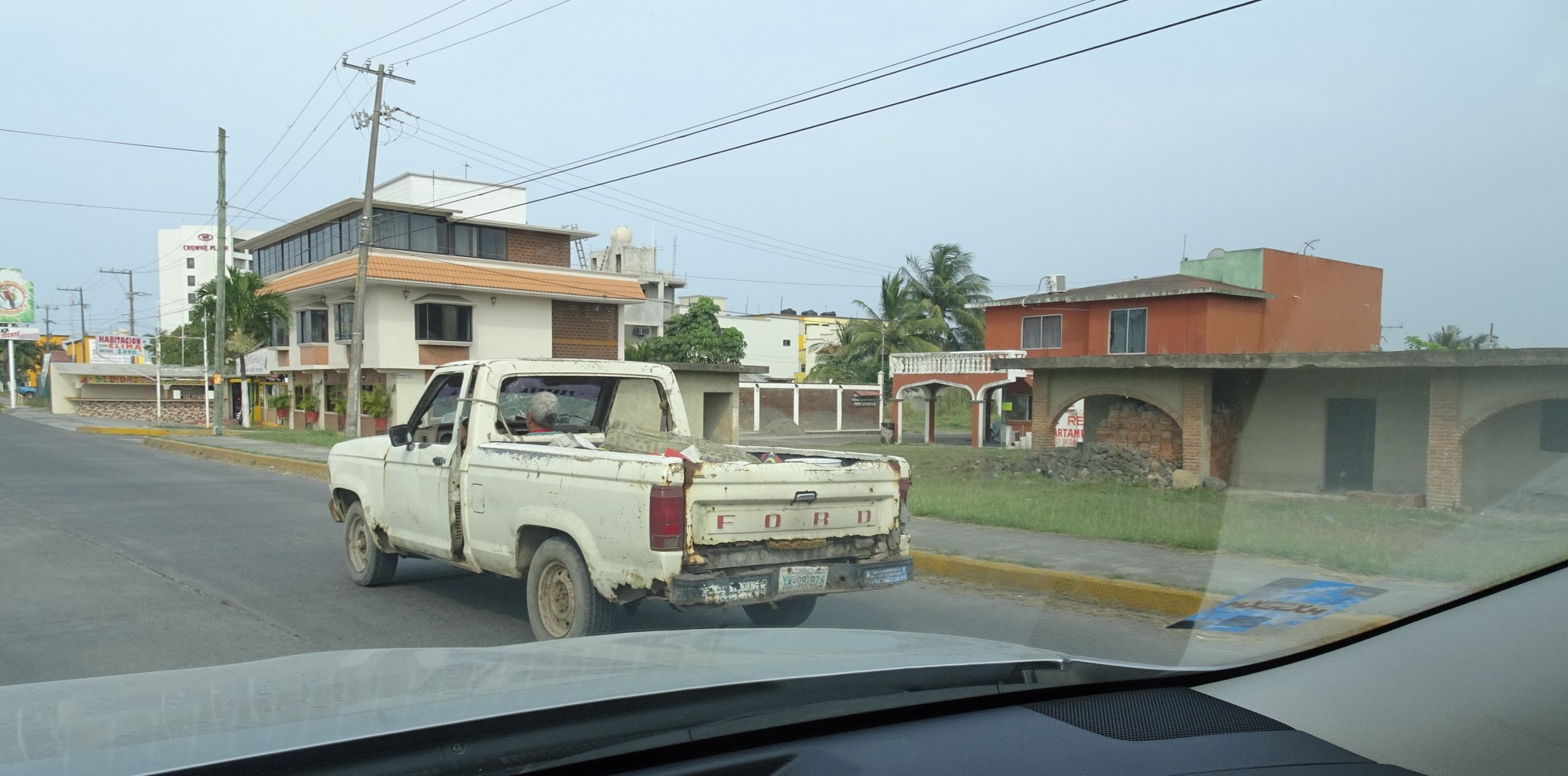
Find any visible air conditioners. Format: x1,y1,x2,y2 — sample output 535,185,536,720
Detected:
1046,275,1067,292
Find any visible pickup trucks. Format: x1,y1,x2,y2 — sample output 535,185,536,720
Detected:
328,360,913,644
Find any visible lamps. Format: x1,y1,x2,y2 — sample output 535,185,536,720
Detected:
402,289,410,300
324,373,327,380
362,372,366,380
320,292,326,303
490,297,497,306
351,285,355,297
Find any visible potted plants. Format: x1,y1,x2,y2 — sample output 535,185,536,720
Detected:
266,391,292,420
326,383,348,427
360,382,396,431
297,380,323,423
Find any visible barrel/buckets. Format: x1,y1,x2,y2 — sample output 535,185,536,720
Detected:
881,423,895,444
785,458,843,468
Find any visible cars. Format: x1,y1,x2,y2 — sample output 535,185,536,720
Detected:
16,385,39,400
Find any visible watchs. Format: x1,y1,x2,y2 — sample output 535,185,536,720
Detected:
460,436,467,442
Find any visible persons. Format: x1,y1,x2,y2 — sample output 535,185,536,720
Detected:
460,391,568,449
